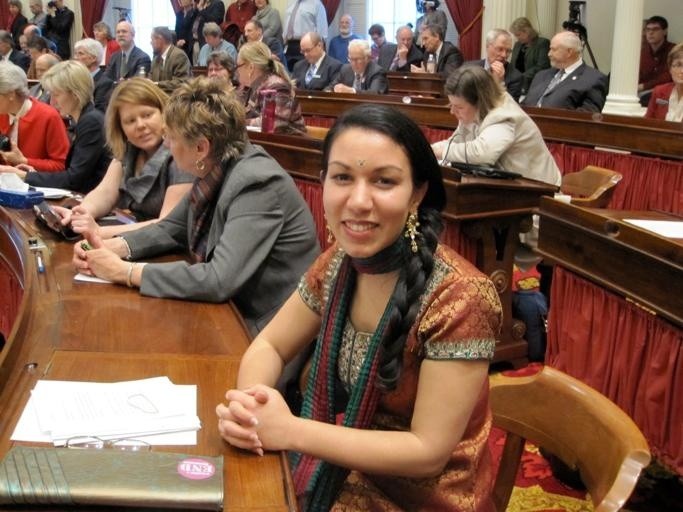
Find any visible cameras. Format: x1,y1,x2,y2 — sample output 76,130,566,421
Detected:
47,1,55,7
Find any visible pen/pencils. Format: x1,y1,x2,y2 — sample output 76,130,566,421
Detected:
80,241,92,251
38,256,44,272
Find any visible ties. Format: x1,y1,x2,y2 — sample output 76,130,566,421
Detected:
120,54,126,78
537,69,565,105
356,74,360,89
286,0,302,41
305,64,316,86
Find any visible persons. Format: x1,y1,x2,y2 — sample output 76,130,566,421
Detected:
217,102,504,512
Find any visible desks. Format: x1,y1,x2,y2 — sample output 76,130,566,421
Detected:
0,63,681,512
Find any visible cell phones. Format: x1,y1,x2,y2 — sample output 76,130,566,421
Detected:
34,200,65,232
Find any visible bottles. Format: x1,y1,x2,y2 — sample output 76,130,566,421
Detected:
427,54,435,74
260,89,276,134
139,67,145,78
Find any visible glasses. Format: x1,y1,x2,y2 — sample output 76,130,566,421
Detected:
299,46,316,54
55,436,151,451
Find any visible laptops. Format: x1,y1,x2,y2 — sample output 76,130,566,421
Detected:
452,162,523,182
